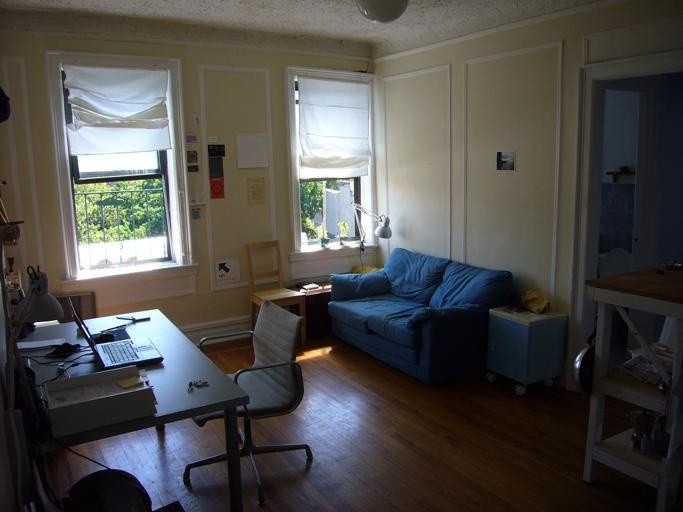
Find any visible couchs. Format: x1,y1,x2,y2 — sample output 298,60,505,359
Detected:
327,247,513,388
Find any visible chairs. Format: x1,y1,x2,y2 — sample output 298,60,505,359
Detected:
245,239,307,348
180,298,313,509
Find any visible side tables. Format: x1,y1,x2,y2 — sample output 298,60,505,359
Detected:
289,284,333,337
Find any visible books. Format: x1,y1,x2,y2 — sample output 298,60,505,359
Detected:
300,285,322,293
296,281,319,290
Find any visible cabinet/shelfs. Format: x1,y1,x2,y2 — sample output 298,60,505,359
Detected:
584,264,683,512
486,307,568,397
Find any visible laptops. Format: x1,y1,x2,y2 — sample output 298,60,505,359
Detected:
66,295,164,370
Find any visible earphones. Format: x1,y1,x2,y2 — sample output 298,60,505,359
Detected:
57,367,65,373
59,363,64,367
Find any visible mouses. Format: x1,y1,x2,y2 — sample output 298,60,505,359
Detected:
94,334,114,343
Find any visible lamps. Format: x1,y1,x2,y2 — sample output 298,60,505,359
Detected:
355,0,409,24
8,264,65,511
350,203,392,273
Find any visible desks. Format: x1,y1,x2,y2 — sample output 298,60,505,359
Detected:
16,309,250,512
